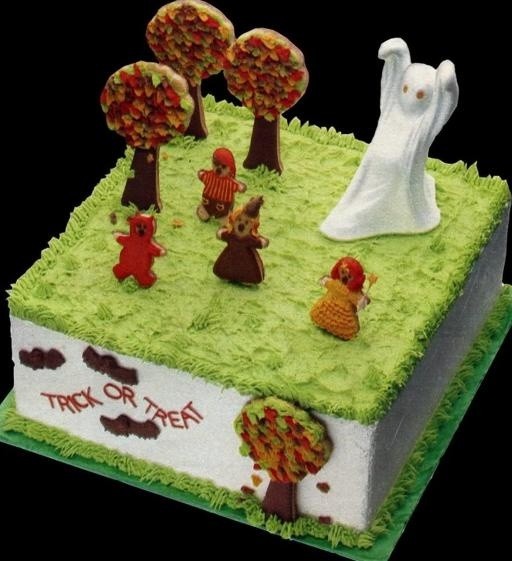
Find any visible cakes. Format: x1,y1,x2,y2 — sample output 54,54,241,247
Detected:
0,1,512,560
